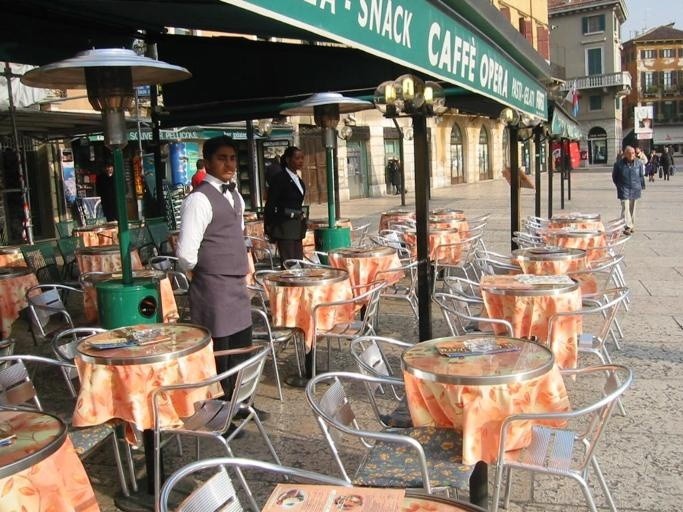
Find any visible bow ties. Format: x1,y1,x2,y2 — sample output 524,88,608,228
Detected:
222,182,236,193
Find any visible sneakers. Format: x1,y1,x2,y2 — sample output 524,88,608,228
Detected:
624,228,633,234
233,406,270,420
222,422,245,438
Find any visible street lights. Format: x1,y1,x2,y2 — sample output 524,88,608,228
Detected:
374,71,445,343
19,46,193,325
279,89,372,268
497,105,581,252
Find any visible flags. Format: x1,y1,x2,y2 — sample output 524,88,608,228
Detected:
565,80,578,116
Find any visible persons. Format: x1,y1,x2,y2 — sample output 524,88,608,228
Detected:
385,158,407,195
191,158,204,188
612,144,675,182
177,137,273,440
265,154,280,184
263,146,306,270
95,165,121,222
611,146,645,235
